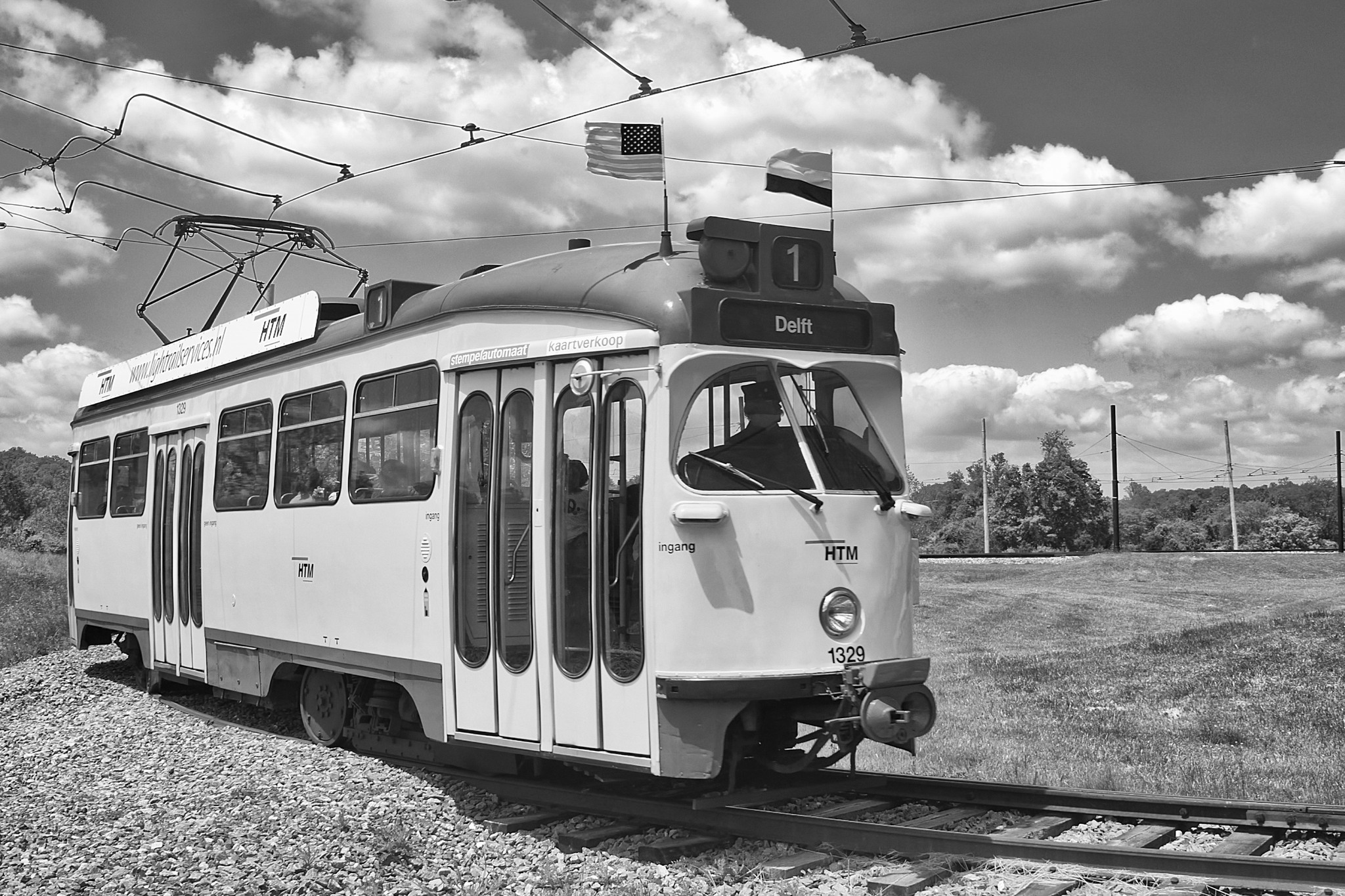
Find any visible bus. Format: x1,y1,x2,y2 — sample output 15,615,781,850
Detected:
66,213,934,792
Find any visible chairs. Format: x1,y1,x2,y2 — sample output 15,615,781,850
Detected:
355,488,384,499
280,493,297,504
115,504,142,513
245,494,261,506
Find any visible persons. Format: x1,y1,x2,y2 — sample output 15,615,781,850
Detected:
742,380,784,429
565,459,588,552
289,459,419,504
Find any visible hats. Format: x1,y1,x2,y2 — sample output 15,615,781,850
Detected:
741,380,782,400
